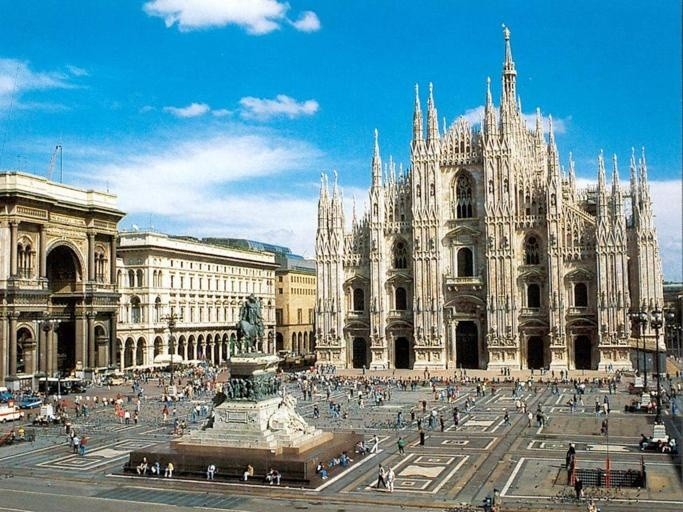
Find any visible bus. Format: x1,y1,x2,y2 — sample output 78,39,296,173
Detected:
37,376,73,395
4,375,21,395
63,376,91,394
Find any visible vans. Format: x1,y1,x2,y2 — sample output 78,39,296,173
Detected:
0,404,25,424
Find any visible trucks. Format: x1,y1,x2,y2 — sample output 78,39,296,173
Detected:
0,385,13,403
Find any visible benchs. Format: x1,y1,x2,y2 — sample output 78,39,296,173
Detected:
309,445,375,491
122,463,311,488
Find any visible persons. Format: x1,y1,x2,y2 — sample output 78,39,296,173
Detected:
242,294,264,326
477,495,494,512
491,488,503,512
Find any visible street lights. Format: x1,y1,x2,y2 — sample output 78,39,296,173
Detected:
625,310,641,376
666,324,682,362
167,319,176,386
647,300,668,442
40,309,53,405
638,309,649,386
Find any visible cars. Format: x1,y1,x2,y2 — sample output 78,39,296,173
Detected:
21,397,43,410
